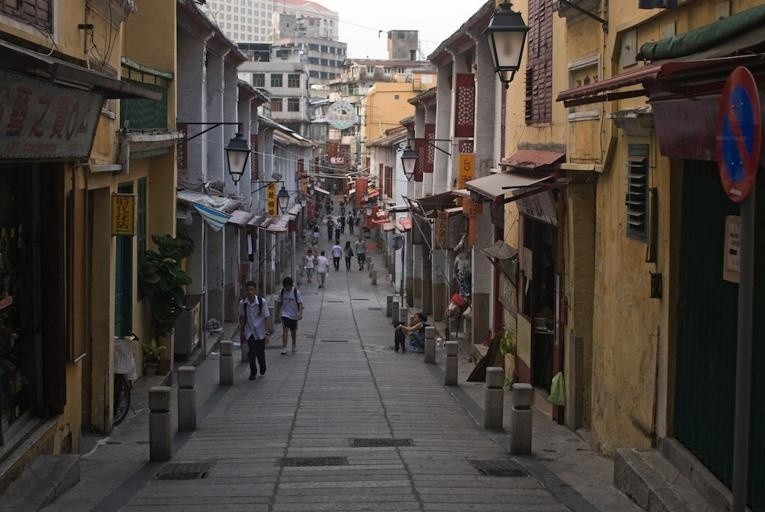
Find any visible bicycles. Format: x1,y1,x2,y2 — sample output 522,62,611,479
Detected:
111,326,140,426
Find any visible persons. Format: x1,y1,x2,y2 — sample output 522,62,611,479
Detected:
273,277,302,355
300,188,370,289
394,313,432,355
239,280,275,381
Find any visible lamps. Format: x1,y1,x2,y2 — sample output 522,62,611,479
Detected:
175,122,251,186
401,137,453,181
251,180,290,215
480,0,609,90
294,189,308,209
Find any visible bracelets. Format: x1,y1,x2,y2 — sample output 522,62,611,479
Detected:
265,328,272,330
266,330,272,333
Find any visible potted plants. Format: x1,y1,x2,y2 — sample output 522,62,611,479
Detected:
138,233,191,377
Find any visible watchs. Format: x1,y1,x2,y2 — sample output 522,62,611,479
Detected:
265,332,270,336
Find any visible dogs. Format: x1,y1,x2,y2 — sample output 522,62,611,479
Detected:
392,321,406,354
